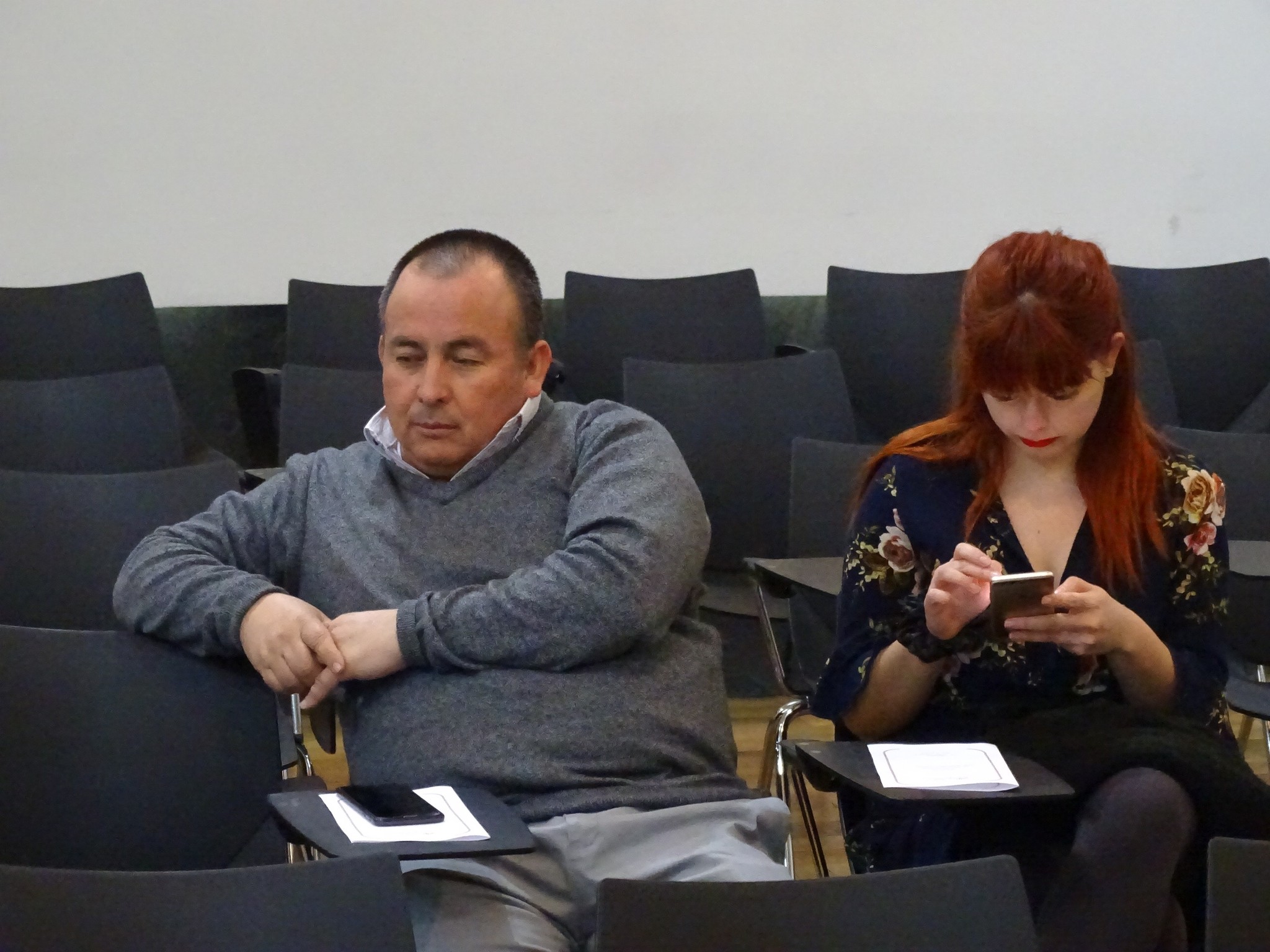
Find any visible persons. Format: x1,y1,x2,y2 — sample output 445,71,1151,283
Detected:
114,229,793,952
811,230,1269,952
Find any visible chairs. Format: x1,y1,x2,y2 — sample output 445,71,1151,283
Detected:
1,260,1269,952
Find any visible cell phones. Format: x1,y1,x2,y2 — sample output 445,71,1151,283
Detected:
336,784,444,826
989,571,1054,639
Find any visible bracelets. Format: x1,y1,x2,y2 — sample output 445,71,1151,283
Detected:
894,604,986,664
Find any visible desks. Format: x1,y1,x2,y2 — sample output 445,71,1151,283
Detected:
264,785,538,860
749,556,844,598
792,741,1074,806
